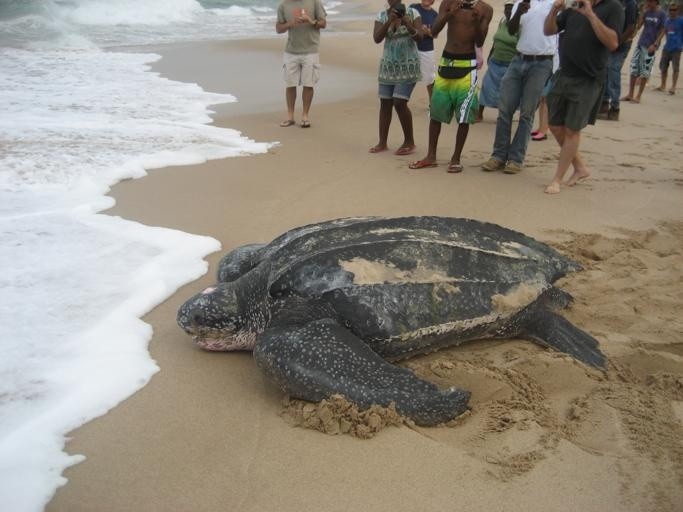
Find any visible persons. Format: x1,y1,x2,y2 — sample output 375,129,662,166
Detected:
275,0,327,129
406,0,682,193
368,0,424,155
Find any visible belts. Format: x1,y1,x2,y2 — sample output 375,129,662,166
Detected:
516,52,553,63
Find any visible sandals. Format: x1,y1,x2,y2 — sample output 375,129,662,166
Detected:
503,159,522,174
480,156,506,171
531,130,548,141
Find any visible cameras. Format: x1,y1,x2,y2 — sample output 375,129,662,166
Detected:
394,4,405,18
462,2,473,10
564,0,580,10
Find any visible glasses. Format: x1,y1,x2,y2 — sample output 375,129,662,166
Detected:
668,9,678,11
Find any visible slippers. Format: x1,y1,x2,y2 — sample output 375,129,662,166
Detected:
279,120,296,127
300,119,311,128
394,143,417,155
408,160,438,169
446,162,463,173
368,145,388,153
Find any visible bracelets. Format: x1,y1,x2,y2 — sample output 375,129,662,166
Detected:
314,19,318,27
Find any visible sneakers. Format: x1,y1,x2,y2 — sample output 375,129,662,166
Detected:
599,101,619,121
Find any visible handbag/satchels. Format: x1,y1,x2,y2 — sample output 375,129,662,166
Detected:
437,64,477,80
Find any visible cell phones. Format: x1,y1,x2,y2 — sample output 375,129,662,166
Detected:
523,0,530,3
